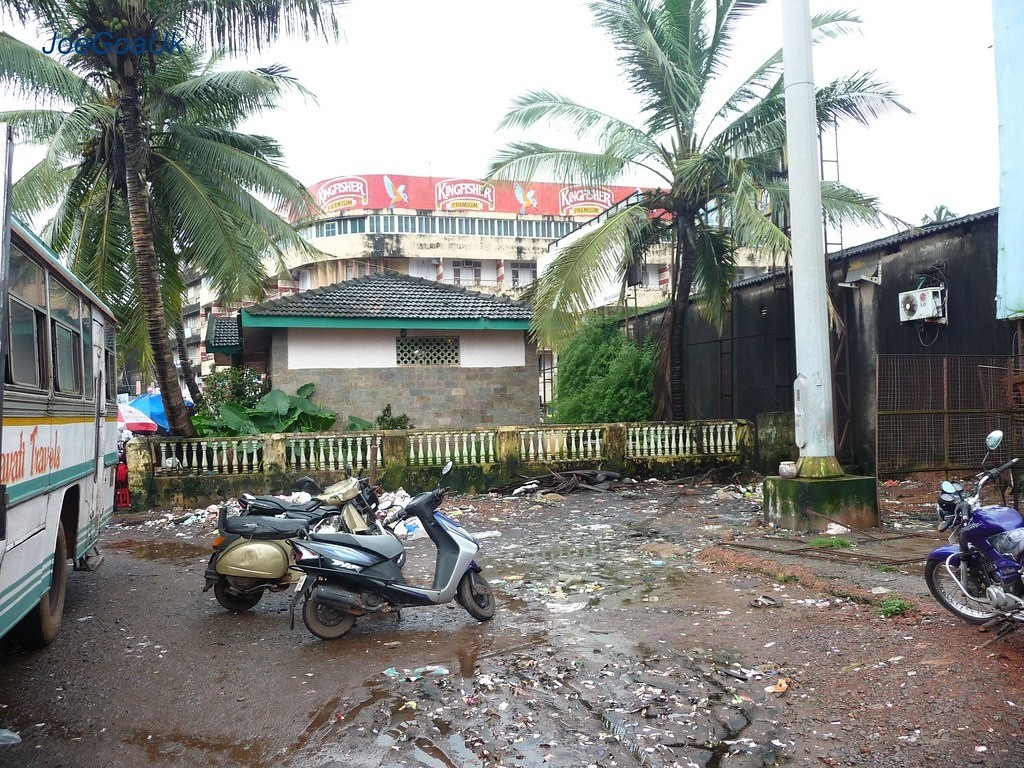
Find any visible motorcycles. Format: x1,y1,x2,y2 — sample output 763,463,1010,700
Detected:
921,428,1024,638
200,463,408,612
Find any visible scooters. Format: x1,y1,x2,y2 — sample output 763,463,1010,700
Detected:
286,460,498,641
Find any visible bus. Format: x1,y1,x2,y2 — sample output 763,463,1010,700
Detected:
0,211,120,651
